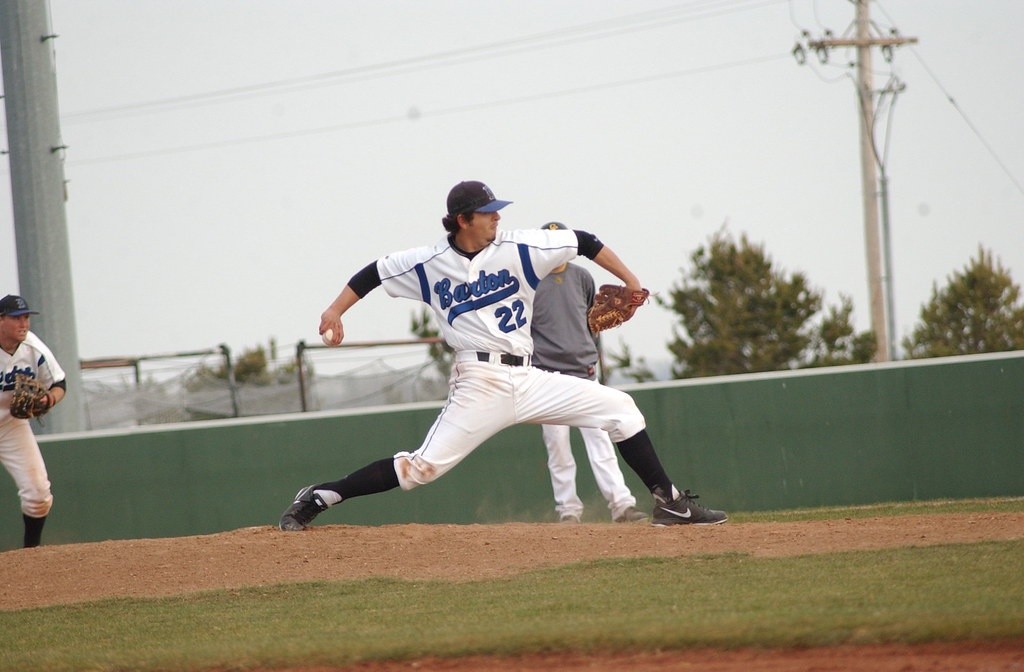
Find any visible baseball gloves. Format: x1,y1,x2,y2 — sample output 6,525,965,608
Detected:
9,373,56,429
586,283,659,339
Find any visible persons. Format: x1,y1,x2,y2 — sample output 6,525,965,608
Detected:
281,182,727,533
532,222,648,522
0,295,66,548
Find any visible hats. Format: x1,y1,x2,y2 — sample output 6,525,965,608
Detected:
541,222,566,230
447,181,513,212
0,294,39,316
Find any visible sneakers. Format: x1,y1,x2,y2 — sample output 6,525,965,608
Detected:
616,507,650,523
651,485,728,526
280,483,330,531
561,516,578,524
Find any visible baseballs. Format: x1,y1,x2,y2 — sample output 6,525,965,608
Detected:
322,328,339,345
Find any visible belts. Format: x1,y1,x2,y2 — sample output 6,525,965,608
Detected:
476,352,530,366
